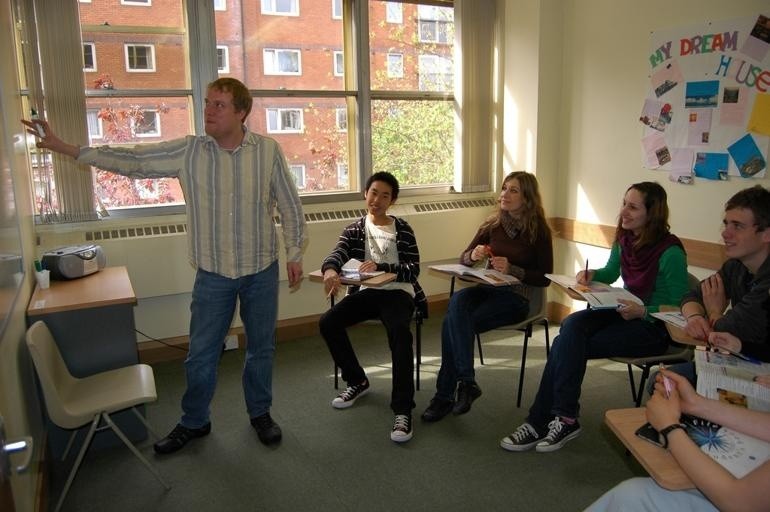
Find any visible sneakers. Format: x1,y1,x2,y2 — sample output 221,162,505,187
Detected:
154,422,211,454
535,416,584,453
331,376,370,409
500,422,546,452
451,380,482,415
390,411,415,442
421,391,456,423
250,412,282,447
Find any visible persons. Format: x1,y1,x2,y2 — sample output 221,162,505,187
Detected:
20,77,310,455
580,367,770,512
646,184,770,397
501,182,691,453
707,332,770,363
320,171,428,442
421,171,561,422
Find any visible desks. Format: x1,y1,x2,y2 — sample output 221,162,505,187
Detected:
27,264,150,466
659,303,711,348
309,269,398,287
554,280,586,301
604,406,698,492
426,267,493,286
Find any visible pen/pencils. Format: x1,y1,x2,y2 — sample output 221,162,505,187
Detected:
586,259,588,280
327,272,342,300
659,361,671,398
716,344,761,365
484,244,497,262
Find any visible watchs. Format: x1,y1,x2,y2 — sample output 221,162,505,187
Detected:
659,424,688,448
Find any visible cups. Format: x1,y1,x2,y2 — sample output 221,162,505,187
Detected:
34,270,51,291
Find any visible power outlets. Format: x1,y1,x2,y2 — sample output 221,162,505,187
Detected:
222,335,239,351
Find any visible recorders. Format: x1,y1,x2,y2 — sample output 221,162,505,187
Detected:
41,244,106,281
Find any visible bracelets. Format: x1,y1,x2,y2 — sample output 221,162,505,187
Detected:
687,313,703,318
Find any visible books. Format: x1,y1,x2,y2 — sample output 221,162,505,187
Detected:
426,264,521,287
342,257,385,282
634,412,770,479
694,349,770,411
649,312,687,329
544,273,645,311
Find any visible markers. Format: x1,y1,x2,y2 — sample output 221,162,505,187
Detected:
34,260,43,274
31,108,45,138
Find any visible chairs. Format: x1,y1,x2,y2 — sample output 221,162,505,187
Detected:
605,273,703,407
26,320,171,512
476,287,551,409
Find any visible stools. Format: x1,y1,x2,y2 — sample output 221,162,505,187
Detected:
334,305,423,391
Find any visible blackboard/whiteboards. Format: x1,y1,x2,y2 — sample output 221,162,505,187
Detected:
0,80,27,342
